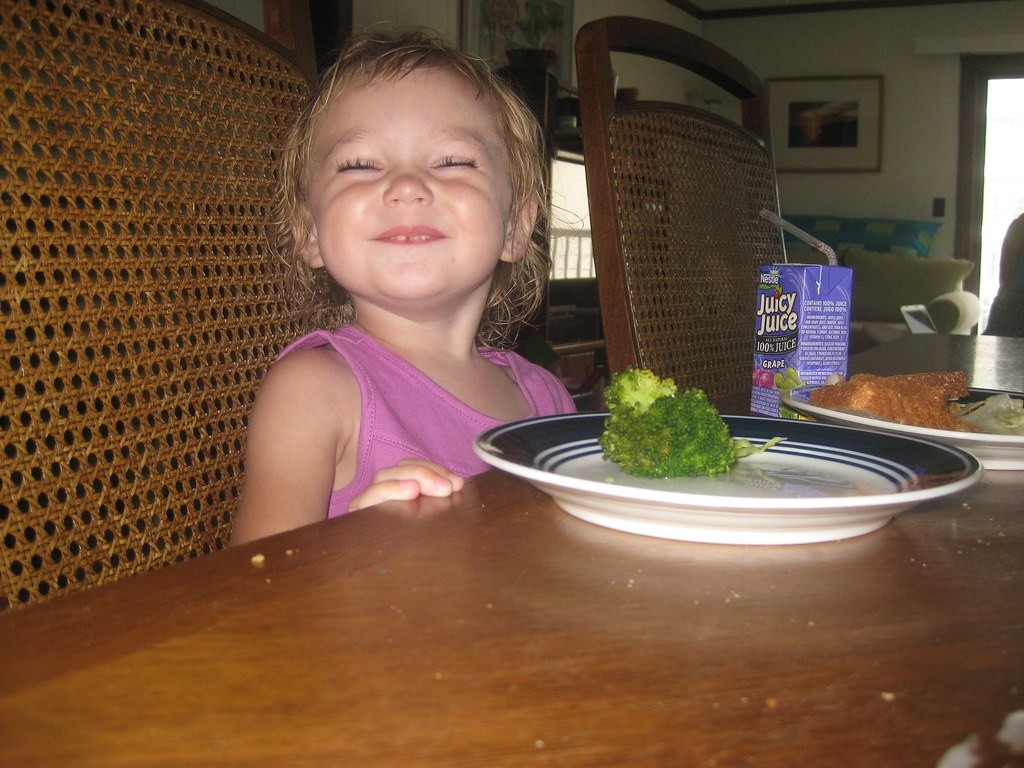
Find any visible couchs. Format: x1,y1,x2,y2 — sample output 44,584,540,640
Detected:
839,246,980,357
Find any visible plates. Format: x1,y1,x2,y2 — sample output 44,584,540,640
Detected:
778,384,1024,471
471,412,984,548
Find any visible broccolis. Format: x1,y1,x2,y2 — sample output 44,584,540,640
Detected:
597,364,741,479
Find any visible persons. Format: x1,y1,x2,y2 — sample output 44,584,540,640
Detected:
225,32,579,548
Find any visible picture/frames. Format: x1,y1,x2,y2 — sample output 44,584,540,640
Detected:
763,74,884,174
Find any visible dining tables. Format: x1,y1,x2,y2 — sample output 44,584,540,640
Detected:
0,333,1024,768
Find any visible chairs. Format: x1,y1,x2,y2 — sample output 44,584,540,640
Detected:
0,0,359,618
575,15,789,415
